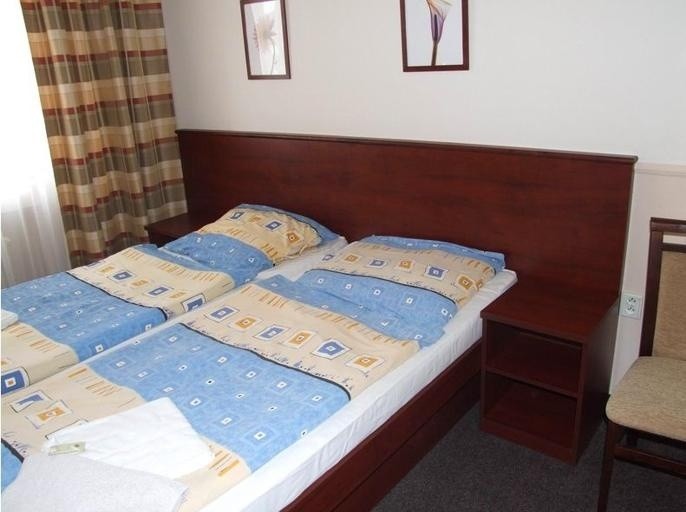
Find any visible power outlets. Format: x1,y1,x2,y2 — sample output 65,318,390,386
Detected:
620,293,644,320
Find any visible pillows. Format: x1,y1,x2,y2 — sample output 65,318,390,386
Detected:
167,201,340,281
304,235,505,326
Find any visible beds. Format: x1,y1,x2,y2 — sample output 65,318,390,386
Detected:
1,203,518,511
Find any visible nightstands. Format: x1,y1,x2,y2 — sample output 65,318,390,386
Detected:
479,275,622,468
143,211,221,247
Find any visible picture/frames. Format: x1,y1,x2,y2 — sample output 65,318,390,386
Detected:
400,0,469,72
240,0,291,80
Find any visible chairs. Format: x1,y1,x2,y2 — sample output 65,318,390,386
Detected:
596,216,686,511
